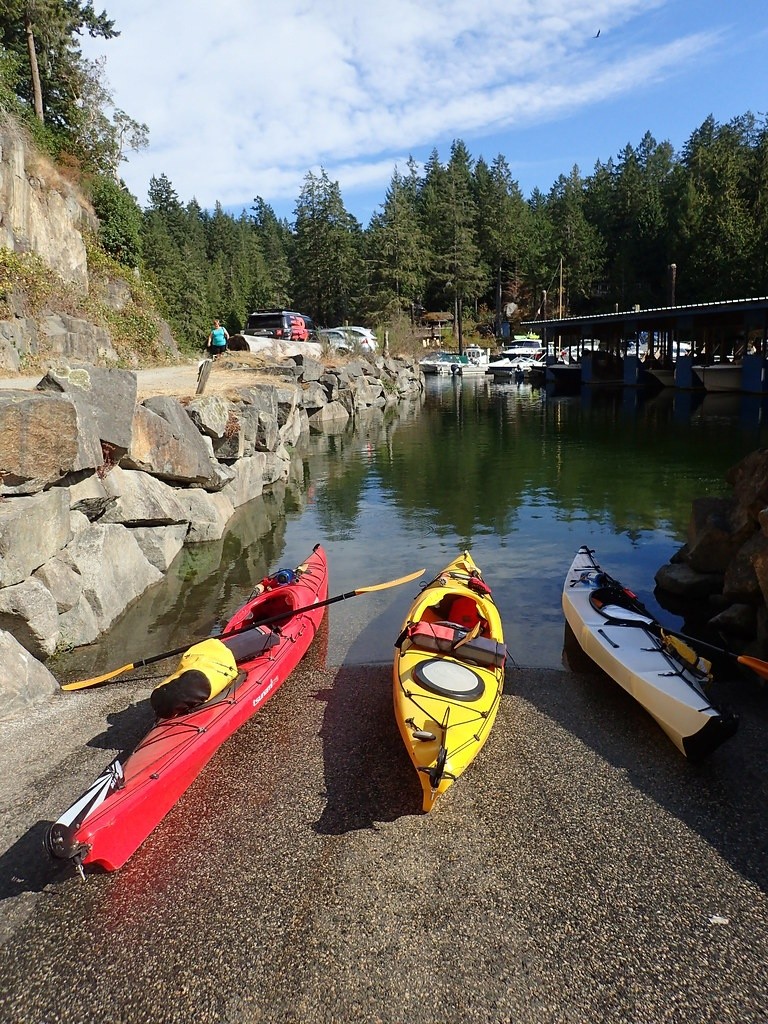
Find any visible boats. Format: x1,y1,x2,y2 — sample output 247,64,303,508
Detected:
43,543,328,883
391,547,508,817
560,544,743,761
419,257,768,393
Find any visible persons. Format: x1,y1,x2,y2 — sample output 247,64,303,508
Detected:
208,319,229,355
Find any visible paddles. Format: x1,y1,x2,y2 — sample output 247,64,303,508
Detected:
58,566,428,692
601,603,768,679
453,618,488,650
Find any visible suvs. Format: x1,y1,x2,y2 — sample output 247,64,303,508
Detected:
333,325,381,356
243,308,322,343
319,330,356,355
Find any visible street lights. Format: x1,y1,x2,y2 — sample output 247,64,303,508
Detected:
670,264,676,306
